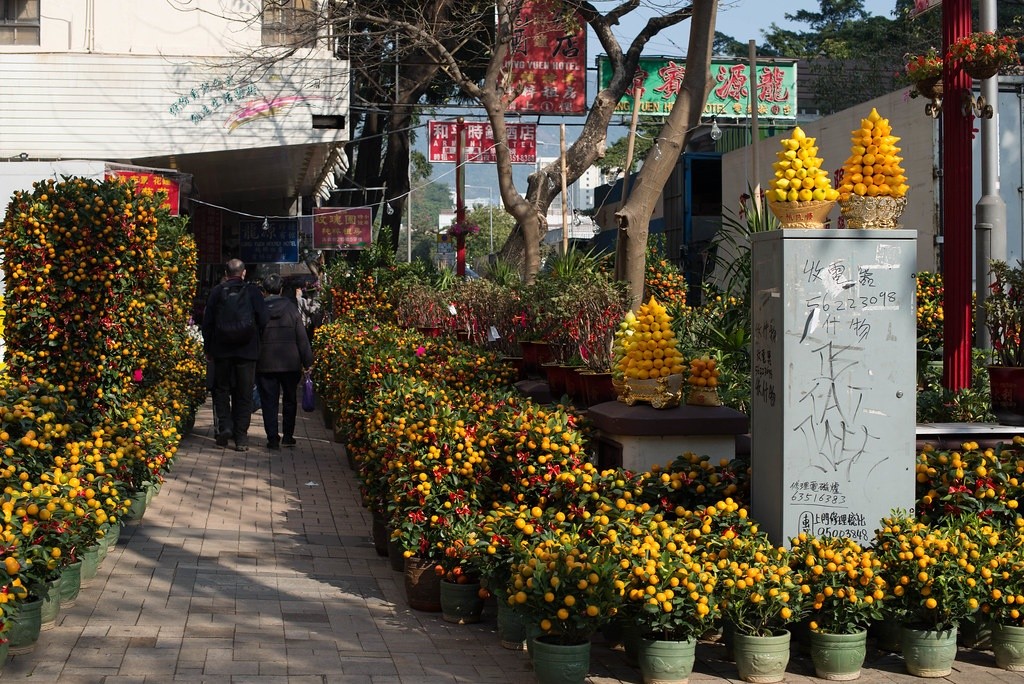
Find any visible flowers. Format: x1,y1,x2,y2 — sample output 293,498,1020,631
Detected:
976,256,1023,367
942,29,1019,66
892,46,944,102
396,270,628,372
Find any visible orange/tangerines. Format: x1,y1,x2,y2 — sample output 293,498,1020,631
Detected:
0,178,208,617
311,248,1024,630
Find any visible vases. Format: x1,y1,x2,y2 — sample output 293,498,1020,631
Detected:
413,325,617,408
986,363,1024,427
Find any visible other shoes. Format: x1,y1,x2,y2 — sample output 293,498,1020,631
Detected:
216,426,234,448
237,443,249,451
282,438,297,444
267,442,279,449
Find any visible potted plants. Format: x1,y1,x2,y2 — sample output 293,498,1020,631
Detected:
331,376,1024,683
0,375,199,670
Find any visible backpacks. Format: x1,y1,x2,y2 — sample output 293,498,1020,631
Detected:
217,282,255,344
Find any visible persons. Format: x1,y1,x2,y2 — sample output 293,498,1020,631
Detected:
202,276,319,394
201,259,258,451
254,274,314,449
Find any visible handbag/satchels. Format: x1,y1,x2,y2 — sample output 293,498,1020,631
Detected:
252,387,262,412
303,372,314,411
